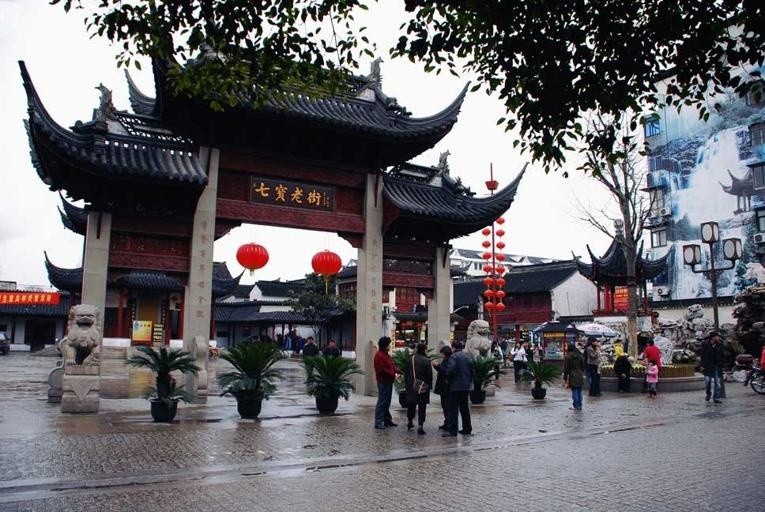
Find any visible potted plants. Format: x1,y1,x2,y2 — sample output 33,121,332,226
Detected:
299,353,365,414
392,348,441,409
123,345,202,422
520,360,566,399
218,342,288,419
469,348,504,404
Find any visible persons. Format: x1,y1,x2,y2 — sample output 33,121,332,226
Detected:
487,336,603,410
302,336,320,386
645,359,659,399
322,339,339,362
699,331,725,404
373,336,400,429
760,347,765,380
614,339,625,361
441,338,473,437
284,330,297,350
614,356,633,393
431,345,453,431
640,339,662,393
404,343,433,435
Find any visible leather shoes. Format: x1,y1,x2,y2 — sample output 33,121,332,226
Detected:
375,421,397,429
439,426,471,437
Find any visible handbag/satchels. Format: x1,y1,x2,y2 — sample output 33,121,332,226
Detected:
414,380,430,394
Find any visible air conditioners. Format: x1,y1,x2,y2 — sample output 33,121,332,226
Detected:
646,210,658,218
656,287,669,296
659,207,671,216
754,233,765,243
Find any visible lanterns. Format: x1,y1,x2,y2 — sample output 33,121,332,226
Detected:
312,249,342,282
482,217,506,317
236,242,269,275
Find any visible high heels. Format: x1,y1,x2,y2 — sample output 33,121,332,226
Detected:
407,423,414,430
417,429,426,434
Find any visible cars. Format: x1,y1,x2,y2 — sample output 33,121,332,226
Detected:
0,331,11,355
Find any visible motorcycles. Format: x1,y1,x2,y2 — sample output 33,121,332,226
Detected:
735,353,765,395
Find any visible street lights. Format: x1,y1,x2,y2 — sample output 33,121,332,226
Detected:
681,221,745,335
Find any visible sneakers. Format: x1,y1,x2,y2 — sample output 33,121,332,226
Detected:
706,392,712,401
714,399,722,403
569,407,582,410
